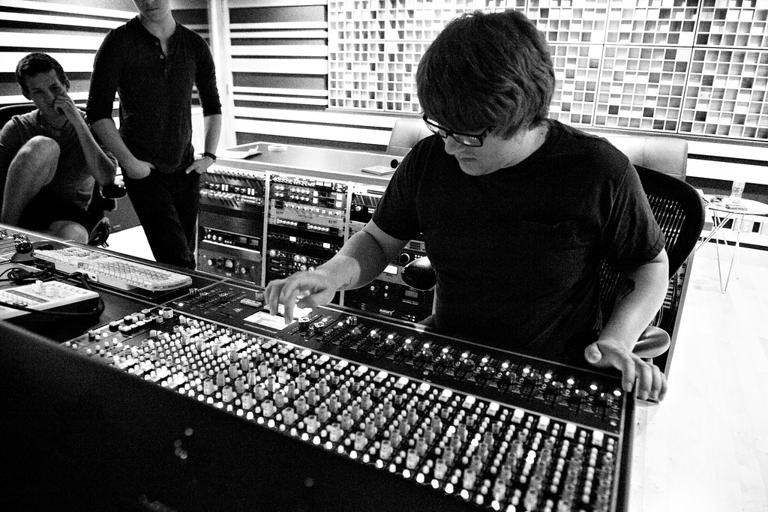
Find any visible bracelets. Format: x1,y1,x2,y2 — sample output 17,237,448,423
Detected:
201,151,216,162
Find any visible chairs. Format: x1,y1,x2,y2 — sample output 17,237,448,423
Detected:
401,164,705,364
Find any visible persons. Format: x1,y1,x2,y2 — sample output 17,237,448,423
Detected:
261,8,671,406
84,1,223,272
0,51,118,245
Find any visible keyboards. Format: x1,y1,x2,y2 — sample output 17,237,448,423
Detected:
34,247,193,294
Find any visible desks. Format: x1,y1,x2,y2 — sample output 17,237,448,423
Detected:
696,193,768,295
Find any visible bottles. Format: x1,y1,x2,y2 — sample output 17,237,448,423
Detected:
731,171,745,206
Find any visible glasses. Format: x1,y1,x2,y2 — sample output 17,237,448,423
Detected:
422,114,493,148
